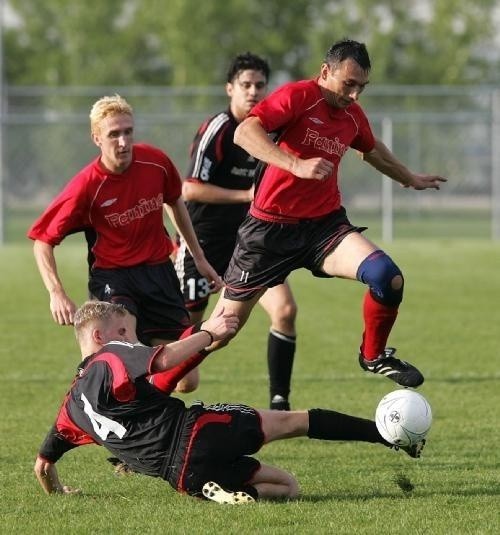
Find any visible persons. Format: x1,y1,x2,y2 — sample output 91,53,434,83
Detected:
34,302,425,508
27,94,224,397
151,38,447,392
173,54,296,410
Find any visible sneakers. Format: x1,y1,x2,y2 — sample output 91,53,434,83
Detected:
200,478,259,507
403,439,426,460
355,345,425,390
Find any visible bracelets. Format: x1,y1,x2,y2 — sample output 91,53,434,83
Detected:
200,330,213,346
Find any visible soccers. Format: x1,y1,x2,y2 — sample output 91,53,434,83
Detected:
375,388,435,449
